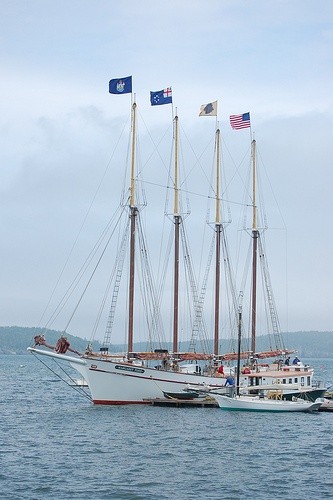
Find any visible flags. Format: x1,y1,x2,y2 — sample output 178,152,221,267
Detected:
150,87,172,106
108,76,132,95
230,112,250,131
198,100,217,116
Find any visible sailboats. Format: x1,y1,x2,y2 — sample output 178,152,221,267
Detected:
26,75,333,407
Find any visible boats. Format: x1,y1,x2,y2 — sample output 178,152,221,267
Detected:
205,384,329,413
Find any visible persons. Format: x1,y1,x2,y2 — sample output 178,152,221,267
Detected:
285,357,290,366
224,374,234,399
219,365,223,373
293,356,300,365
273,359,279,364
244,367,250,374
